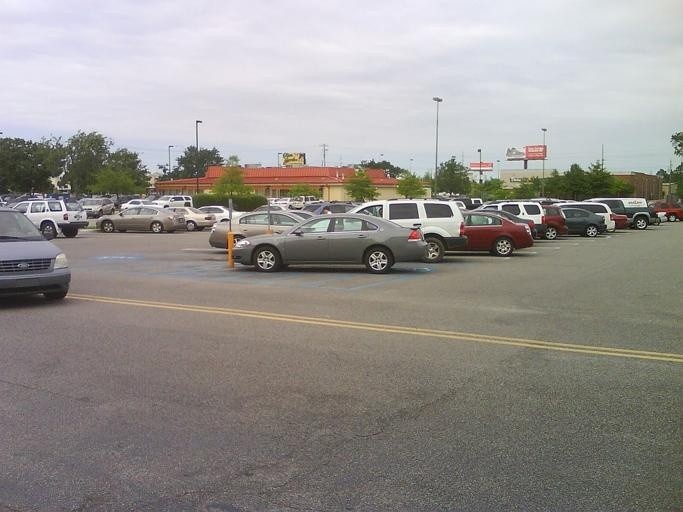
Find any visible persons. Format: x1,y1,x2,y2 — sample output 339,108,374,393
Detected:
322,206,334,214
379,208,382,217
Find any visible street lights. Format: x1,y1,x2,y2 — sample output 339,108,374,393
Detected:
477,148,482,184
195,119,202,194
277,153,281,168
167,145,174,172
432,96,443,192
540,128,548,196
